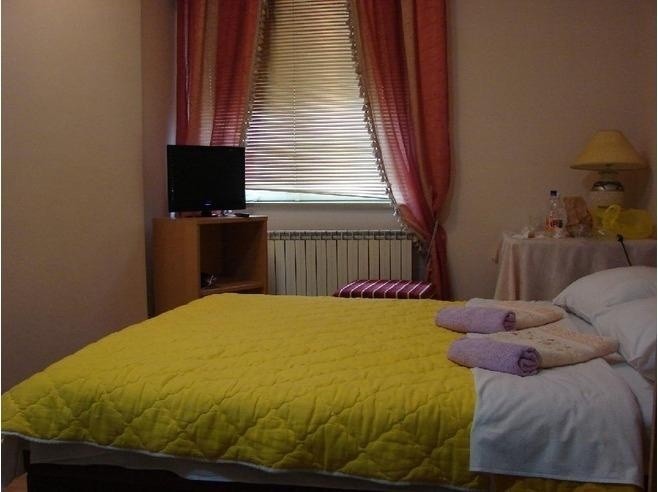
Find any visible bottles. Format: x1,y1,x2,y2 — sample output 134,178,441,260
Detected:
544,190,559,234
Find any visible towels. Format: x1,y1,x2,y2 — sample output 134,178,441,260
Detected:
436,296,617,376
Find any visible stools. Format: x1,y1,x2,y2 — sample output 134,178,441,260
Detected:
331,278,436,300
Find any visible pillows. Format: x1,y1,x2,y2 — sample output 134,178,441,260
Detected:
593,298,656,381
551,267,656,322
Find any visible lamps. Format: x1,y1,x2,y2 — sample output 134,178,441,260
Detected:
569,130,649,229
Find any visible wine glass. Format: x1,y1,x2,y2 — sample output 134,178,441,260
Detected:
549,208,567,239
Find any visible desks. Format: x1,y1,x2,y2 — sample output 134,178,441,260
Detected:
493,231,657,301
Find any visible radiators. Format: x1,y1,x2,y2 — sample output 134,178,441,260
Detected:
266,229,417,296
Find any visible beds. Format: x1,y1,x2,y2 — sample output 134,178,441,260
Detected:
22,294,657,492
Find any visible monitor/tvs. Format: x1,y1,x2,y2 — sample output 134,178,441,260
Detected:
167,144,247,218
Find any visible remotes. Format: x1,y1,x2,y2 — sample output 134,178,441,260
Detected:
234,211,249,217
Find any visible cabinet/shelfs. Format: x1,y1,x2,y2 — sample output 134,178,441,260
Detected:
153,215,269,314
